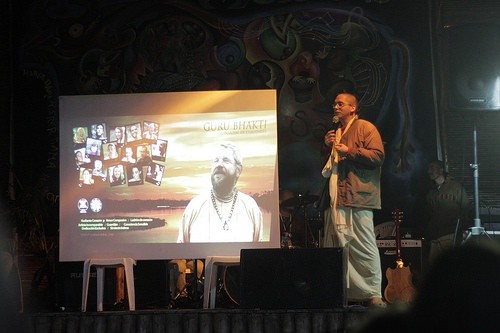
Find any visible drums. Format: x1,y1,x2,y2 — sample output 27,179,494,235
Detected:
278,231,303,248
223,264,240,307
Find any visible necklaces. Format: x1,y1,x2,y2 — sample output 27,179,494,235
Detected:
210,189,238,230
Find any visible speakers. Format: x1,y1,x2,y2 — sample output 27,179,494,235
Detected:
445,22,500,112
239,249,345,308
54,252,115,308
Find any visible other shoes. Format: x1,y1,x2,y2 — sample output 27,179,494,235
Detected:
368,297,387,309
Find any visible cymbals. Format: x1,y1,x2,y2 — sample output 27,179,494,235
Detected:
280,193,320,208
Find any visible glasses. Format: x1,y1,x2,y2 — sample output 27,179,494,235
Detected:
332,102,352,109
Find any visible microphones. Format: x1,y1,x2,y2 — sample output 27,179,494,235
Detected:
331,115,339,134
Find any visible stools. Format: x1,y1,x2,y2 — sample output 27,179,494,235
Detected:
204,256,240,309
82,258,138,312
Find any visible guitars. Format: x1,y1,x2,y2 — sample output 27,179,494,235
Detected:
383,209,418,305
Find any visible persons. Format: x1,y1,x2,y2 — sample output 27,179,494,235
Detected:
178,143,263,242
321,93,388,309
278,190,308,249
72,122,167,188
420,159,468,275
